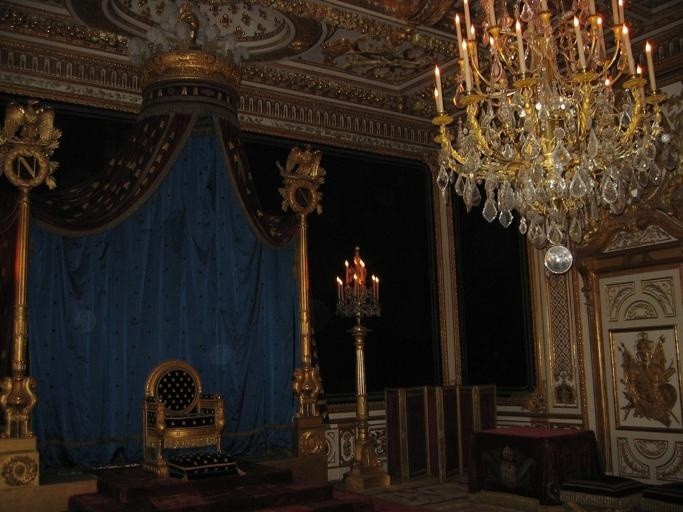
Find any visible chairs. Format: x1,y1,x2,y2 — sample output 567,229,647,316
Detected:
140,360,227,479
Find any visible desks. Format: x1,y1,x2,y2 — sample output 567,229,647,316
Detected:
475,426,595,507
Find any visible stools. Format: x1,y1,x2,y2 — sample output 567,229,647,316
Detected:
559,473,683,512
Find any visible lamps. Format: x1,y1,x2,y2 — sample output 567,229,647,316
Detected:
431,0,676,274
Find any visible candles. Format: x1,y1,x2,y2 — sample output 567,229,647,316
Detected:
336,256,381,305
